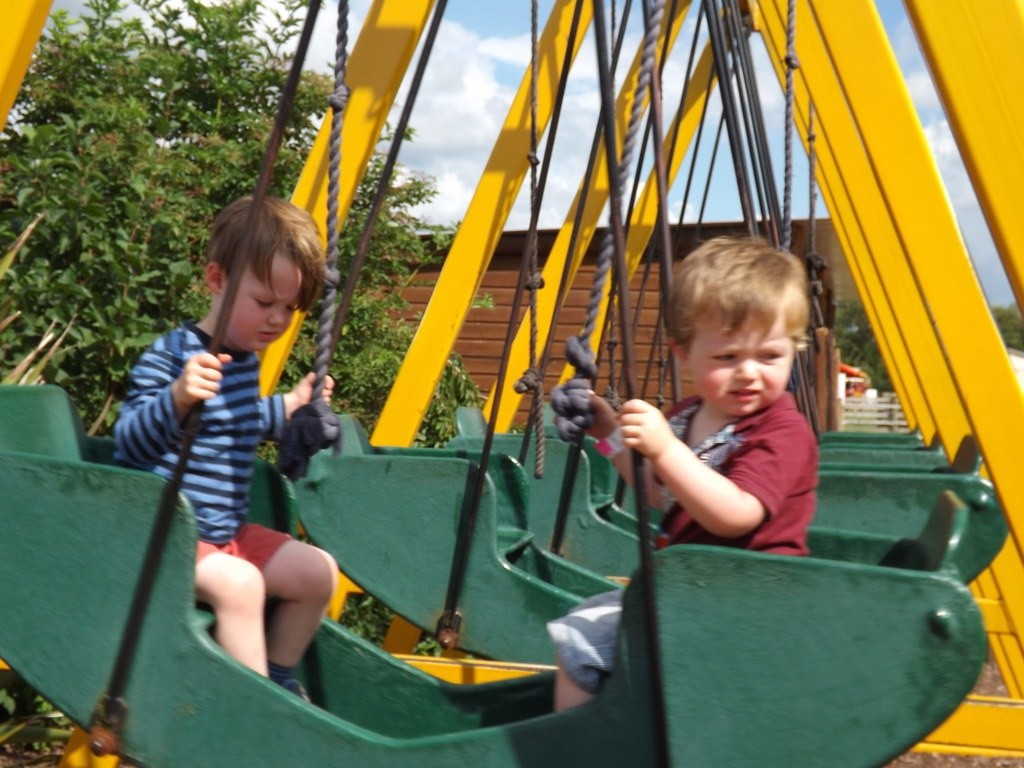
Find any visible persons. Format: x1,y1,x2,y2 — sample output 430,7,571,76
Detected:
115,196,337,698
545,236,819,718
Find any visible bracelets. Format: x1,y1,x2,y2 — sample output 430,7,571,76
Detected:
592,426,630,462
